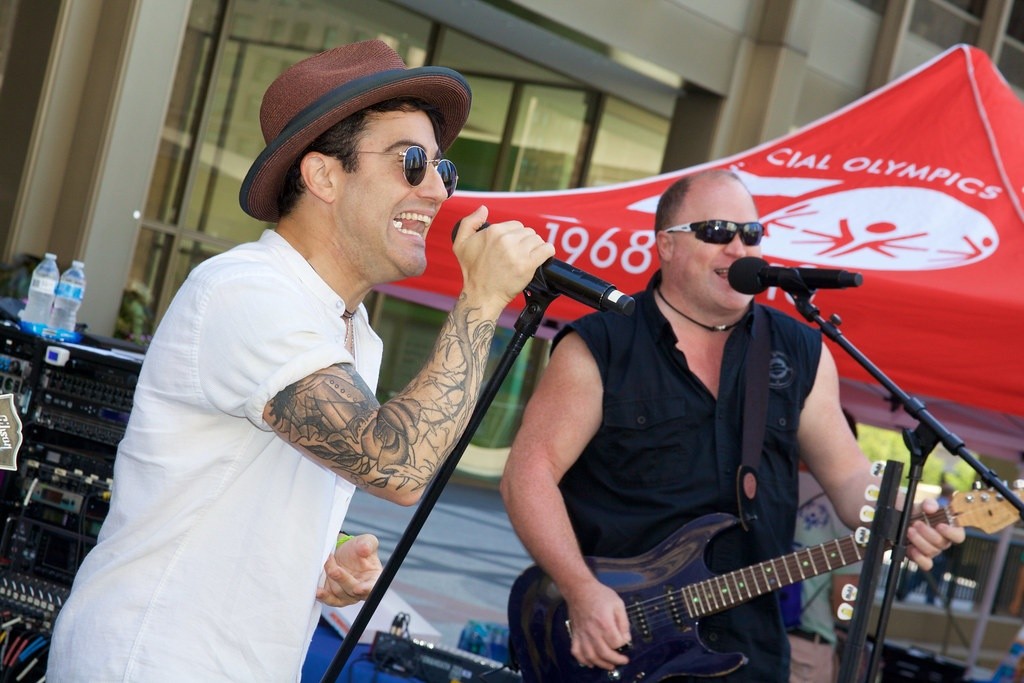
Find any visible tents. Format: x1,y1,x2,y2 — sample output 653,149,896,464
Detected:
373,43,1023,673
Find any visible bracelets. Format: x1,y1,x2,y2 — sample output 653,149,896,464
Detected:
336,536,351,548
339,531,349,537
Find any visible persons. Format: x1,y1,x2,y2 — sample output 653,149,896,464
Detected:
782,408,858,682
500,168,965,683
46,40,554,683
116,282,152,341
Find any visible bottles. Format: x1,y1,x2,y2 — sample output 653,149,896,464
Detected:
22,253,61,323
49,259,86,331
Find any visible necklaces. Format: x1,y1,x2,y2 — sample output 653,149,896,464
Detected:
658,288,741,331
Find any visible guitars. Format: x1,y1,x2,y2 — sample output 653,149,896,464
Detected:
505,478,1023,683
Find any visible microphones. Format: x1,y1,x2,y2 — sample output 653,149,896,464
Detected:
728,257,864,295
451,217,633,317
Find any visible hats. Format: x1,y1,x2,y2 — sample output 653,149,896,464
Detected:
239,39,473,224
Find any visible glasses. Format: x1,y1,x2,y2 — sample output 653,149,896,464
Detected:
344,145,459,199
665,219,765,247
